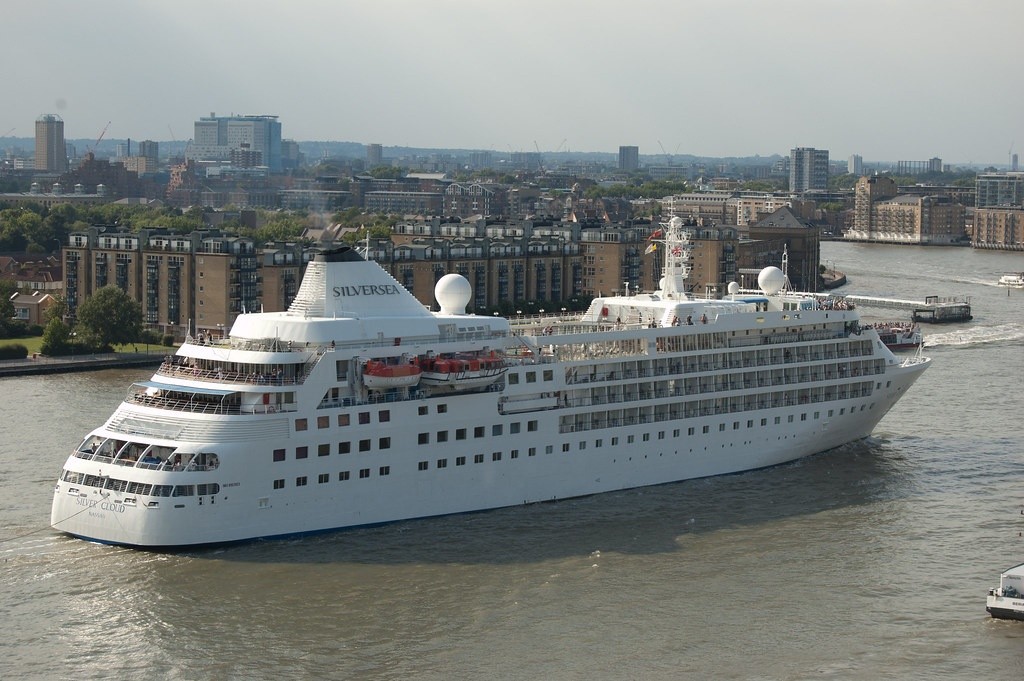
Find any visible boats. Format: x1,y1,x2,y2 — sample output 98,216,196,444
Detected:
417,348,510,392
361,356,422,390
986,563,1023,622
860,320,925,350
912,294,974,324
998,270,1024,289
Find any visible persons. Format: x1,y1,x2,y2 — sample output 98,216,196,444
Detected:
90,330,337,472
815,295,920,338
511,310,708,355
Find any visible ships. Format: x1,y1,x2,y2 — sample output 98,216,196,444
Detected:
49,192,932,550
